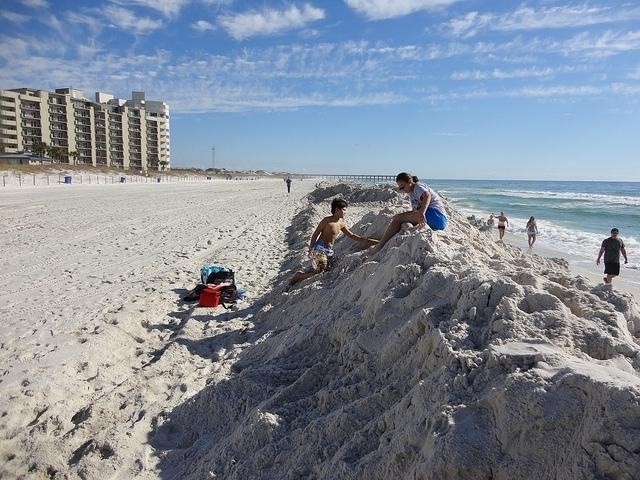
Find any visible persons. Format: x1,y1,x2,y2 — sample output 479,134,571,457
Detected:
369,172,448,256
285,176,292,193
525,215,539,250
596,228,628,283
492,211,510,239
291,196,380,286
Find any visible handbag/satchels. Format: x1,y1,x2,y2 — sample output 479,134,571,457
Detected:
201,265,236,303
